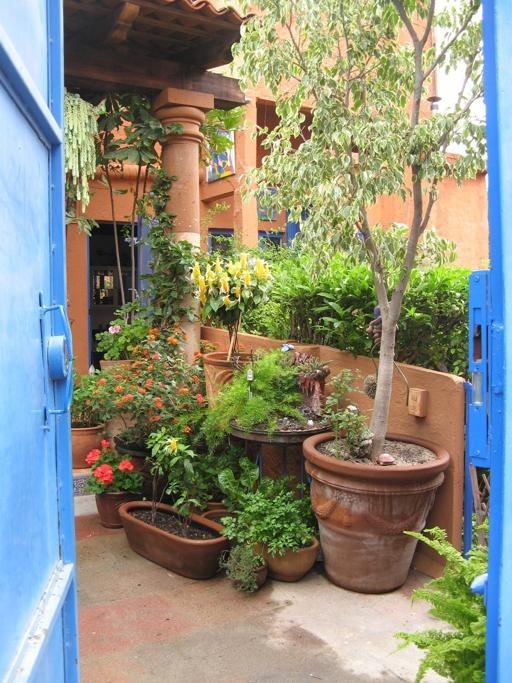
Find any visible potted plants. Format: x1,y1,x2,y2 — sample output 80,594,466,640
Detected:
119,0,488,593
71,364,106,469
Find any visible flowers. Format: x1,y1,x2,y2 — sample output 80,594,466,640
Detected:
86,441,144,496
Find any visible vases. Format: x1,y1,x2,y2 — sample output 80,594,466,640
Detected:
95,492,136,529
